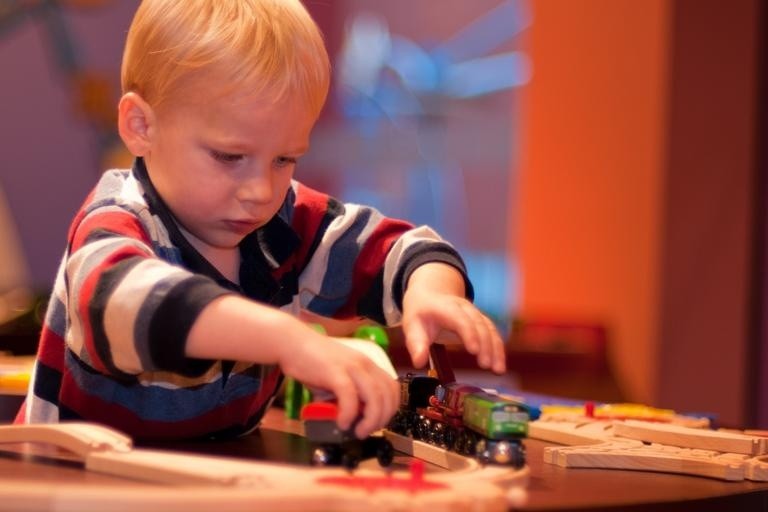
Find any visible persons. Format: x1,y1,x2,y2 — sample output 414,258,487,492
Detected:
12,0,506,471
